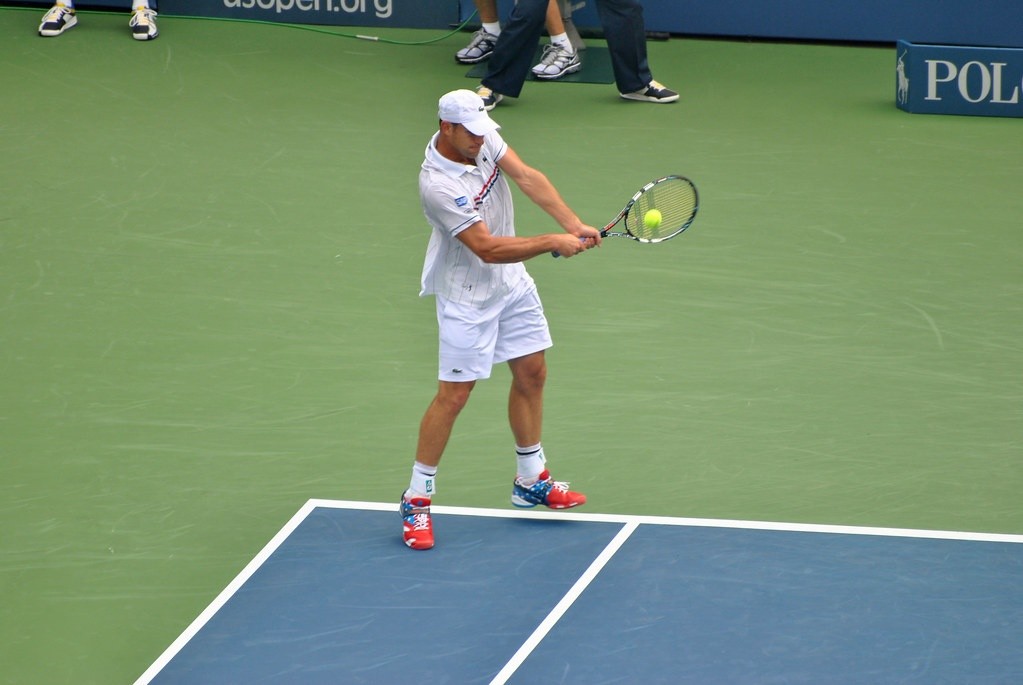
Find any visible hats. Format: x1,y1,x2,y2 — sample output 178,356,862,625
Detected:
439,89,502,136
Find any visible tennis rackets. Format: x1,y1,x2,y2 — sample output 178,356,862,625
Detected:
551,175,700,259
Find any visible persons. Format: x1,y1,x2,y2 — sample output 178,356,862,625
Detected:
401,89,602,550
37,0,157,41
454,0,679,112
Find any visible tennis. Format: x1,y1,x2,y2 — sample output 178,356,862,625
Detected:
644,209,662,228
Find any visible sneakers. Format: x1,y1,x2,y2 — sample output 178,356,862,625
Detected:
531,42,582,78
620,79,680,102
454,26,499,63
399,489,434,549
477,84,503,111
512,469,586,509
39,3,77,36
129,5,159,39
